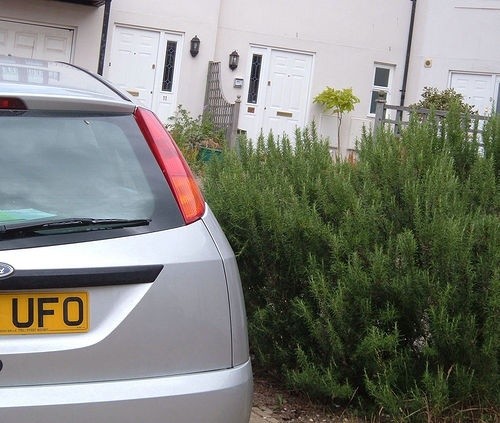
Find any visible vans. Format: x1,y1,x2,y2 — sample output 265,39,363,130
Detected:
1,56,255,422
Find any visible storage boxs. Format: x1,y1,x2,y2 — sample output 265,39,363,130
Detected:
193,143,223,171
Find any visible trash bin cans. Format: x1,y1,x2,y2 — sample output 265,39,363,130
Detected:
196,144,222,167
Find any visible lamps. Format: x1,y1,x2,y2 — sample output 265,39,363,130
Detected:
190,34,200,57
228,50,240,72
